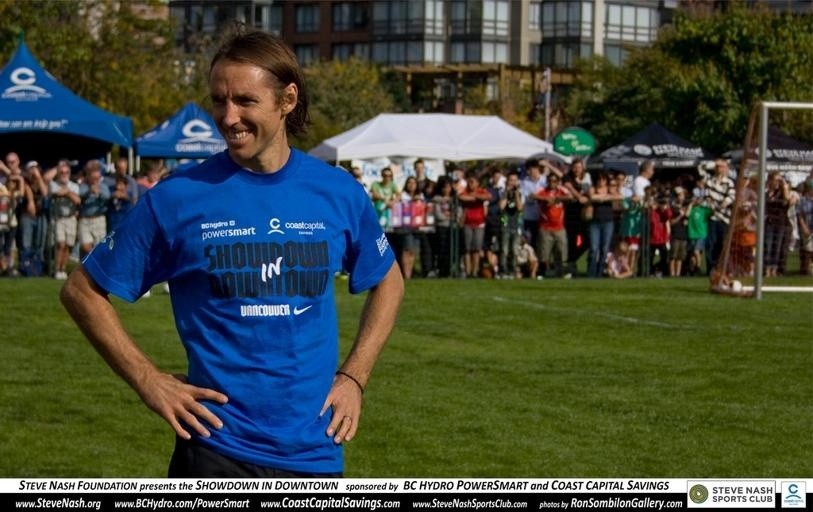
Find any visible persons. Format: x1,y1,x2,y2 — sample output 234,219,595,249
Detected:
60,17,405,478
0,151,813,299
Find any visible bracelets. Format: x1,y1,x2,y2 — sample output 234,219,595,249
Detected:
336,371,363,395
